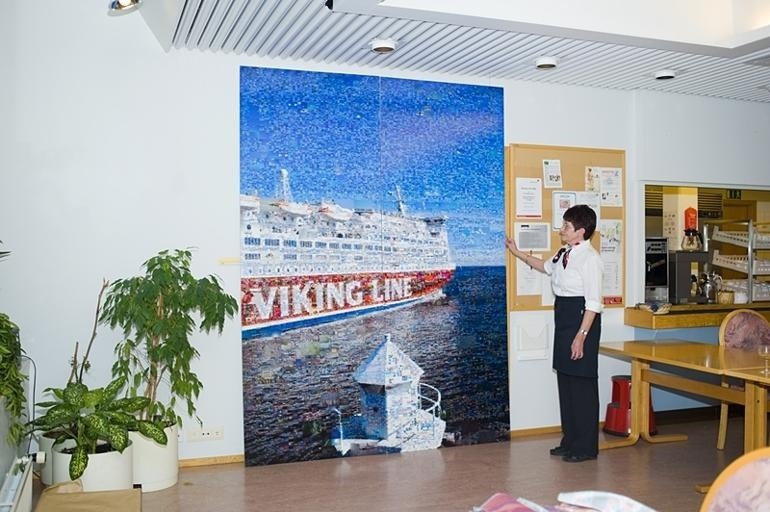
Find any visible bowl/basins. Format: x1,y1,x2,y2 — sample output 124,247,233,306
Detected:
653,303,672,315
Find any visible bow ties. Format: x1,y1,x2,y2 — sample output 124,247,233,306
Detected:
552,247,573,270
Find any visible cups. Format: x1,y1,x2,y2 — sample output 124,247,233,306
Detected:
717,290,735,305
760,360,770,377
758,344,770,359
722,279,770,300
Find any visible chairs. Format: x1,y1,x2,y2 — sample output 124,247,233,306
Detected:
700,447,770,512
717,308,770,450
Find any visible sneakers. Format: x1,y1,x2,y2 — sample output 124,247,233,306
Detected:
547,442,576,458
563,451,599,463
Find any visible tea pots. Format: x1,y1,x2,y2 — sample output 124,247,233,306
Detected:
699,270,723,305
681,228,704,253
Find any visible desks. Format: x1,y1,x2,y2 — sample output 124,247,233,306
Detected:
597,338,770,493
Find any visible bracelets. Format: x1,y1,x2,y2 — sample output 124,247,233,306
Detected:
578,328,588,335
525,254,530,264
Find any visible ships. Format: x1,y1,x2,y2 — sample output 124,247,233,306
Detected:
240,163,455,339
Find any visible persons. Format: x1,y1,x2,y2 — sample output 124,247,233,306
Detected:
503,204,606,462
